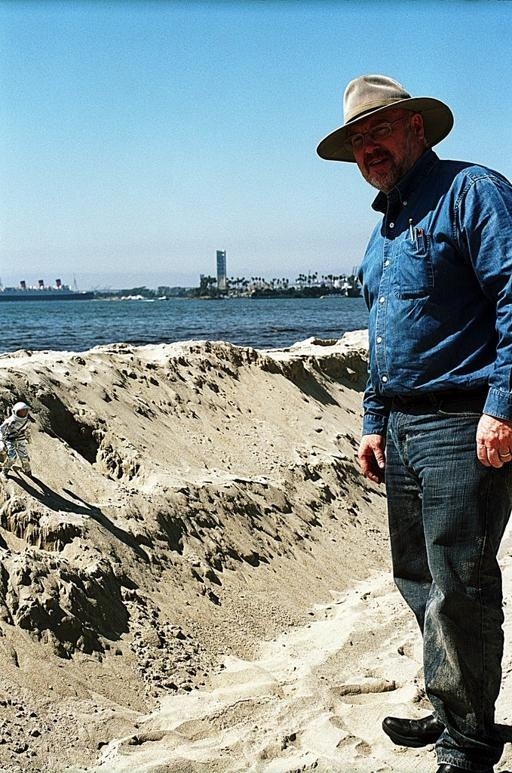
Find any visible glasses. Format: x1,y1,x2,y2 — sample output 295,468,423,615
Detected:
341,115,405,153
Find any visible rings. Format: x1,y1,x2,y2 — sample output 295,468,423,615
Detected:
478,444,486,448
500,453,511,458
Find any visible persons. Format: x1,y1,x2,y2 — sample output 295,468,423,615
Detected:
316,75,512,773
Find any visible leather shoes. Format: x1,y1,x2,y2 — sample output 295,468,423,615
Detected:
381,712,495,773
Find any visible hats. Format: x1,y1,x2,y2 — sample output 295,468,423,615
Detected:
315,74,453,161
